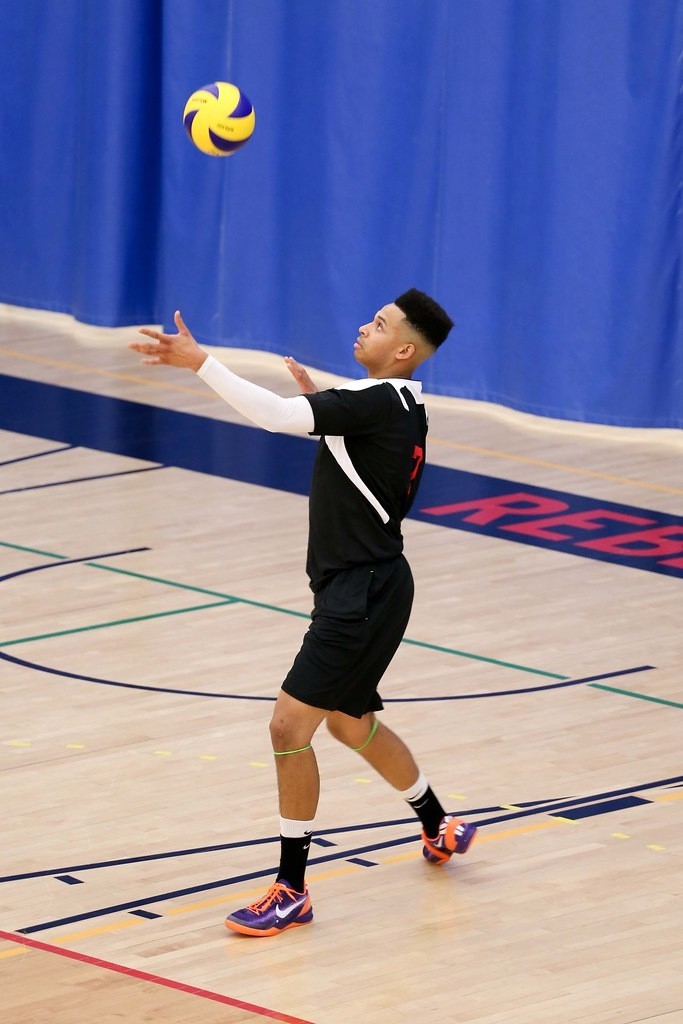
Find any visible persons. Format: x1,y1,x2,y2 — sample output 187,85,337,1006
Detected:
130,288,478,937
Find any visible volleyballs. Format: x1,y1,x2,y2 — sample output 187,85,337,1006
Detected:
184,82,254,158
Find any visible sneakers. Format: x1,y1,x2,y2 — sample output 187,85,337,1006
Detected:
419,815,482,867
229,884,319,934
